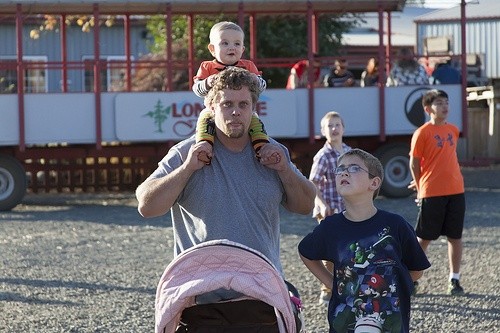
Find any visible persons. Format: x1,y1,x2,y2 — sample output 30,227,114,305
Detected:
297,147,431,333
308,111,352,300
286,51,462,90
408,89,466,297
133,66,316,285
191,21,281,166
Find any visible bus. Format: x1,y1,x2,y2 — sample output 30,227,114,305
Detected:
0,0,470,214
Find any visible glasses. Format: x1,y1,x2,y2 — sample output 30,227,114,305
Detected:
331,164,375,177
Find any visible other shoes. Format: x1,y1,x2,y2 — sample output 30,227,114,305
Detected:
448,278,465,295
411,280,419,296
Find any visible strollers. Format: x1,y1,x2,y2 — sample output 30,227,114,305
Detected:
154,238,306,333
